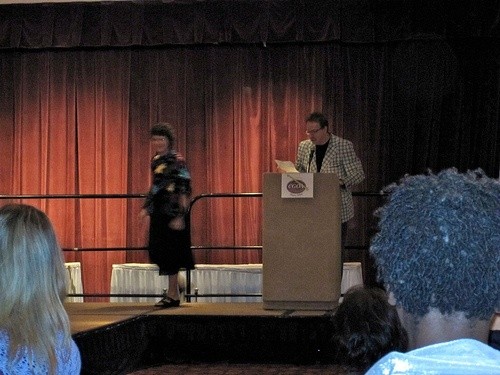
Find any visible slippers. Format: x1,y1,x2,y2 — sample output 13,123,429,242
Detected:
155,296,180,307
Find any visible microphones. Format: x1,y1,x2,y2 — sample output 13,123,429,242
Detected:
308,150,315,173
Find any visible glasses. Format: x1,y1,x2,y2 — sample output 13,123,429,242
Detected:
305,127,325,137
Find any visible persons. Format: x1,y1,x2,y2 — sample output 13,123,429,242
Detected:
331,286,408,372
138,123,194,308
277,112,365,285
0,203,81,375
364,170,500,375
